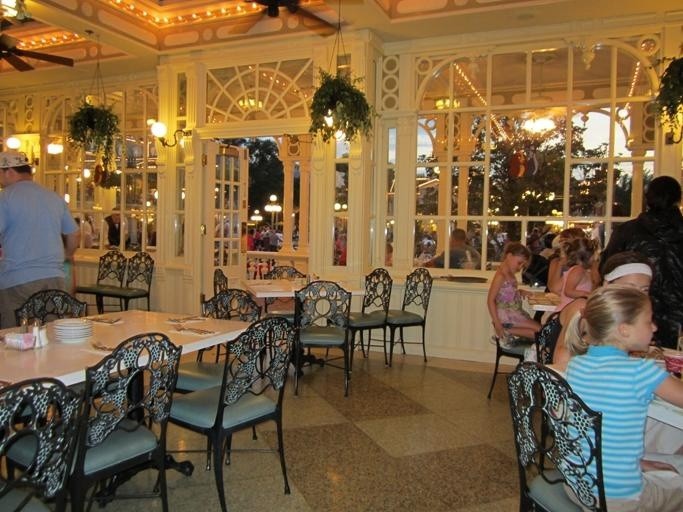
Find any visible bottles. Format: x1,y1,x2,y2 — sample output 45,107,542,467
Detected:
39,320,49,346
20,317,29,334
33,318,43,348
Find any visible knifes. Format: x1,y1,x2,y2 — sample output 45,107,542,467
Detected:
167,318,206,322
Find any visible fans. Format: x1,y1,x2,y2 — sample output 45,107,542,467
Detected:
229,0,339,39
0,33,74,73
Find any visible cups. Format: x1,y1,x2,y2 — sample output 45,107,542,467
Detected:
662,350,683,379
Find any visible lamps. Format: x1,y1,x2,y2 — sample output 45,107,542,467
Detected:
150,120,194,149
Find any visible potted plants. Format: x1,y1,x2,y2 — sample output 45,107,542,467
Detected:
308,67,374,143
66,97,121,178
646,56,683,128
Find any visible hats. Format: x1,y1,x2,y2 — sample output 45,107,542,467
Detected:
0,151,30,168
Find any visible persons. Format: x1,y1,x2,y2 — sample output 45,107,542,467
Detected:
0,150,82,330
487,242,543,351
604,175,683,352
333,227,344,265
99,207,131,252
523,251,654,372
412,221,558,287
546,226,587,292
552,238,601,319
554,283,683,511
239,220,285,252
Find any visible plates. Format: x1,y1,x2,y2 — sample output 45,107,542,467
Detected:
52,318,95,343
